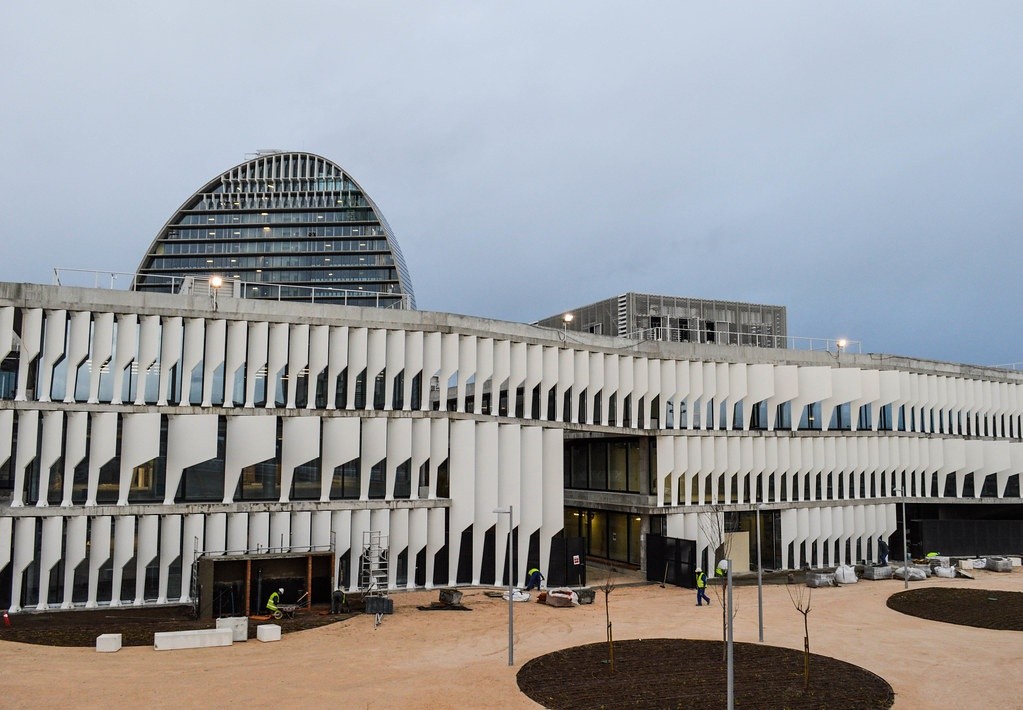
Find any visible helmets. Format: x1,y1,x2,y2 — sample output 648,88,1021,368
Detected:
695,568,702,572
279,588,284,594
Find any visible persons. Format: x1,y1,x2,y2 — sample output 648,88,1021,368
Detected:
879,539,889,565
332,590,344,615
694,568,710,606
524,568,544,591
927,552,940,556
265,588,284,614
715,569,727,577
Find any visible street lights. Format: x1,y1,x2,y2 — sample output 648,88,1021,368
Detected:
755,501,763,642
491,505,515,666
892,486,908,589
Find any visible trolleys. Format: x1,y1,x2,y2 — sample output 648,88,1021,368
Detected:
274,600,312,619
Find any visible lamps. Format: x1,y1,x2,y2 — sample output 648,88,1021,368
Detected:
826,340,846,359
210,276,223,313
557,313,574,343
